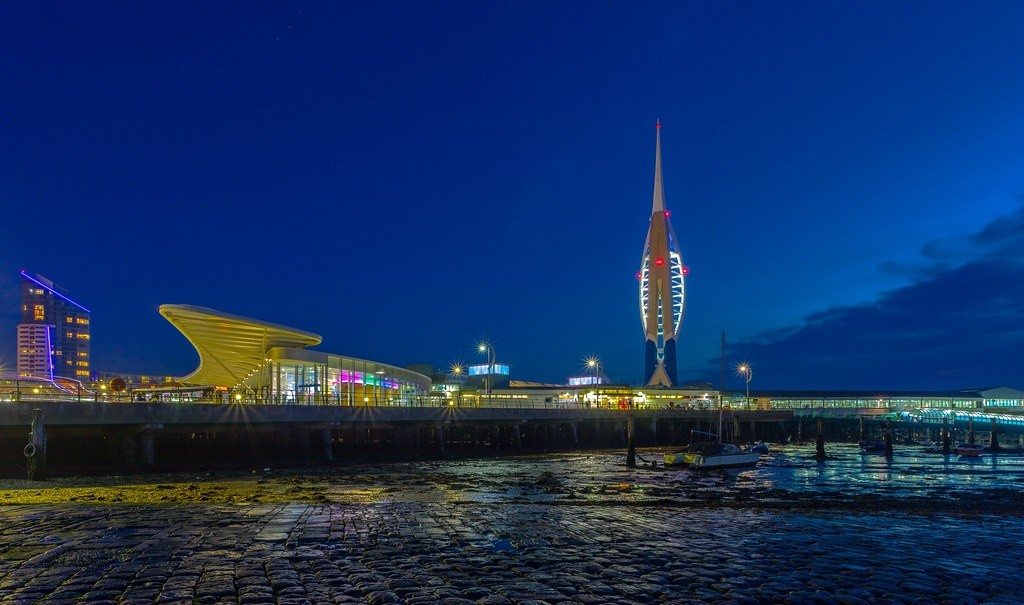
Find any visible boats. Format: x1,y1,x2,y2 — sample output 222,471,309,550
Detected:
663,430,761,471
956,443,986,457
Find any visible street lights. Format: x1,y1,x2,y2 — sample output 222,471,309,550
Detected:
479,340,496,405
740,365,752,408
588,359,599,409
